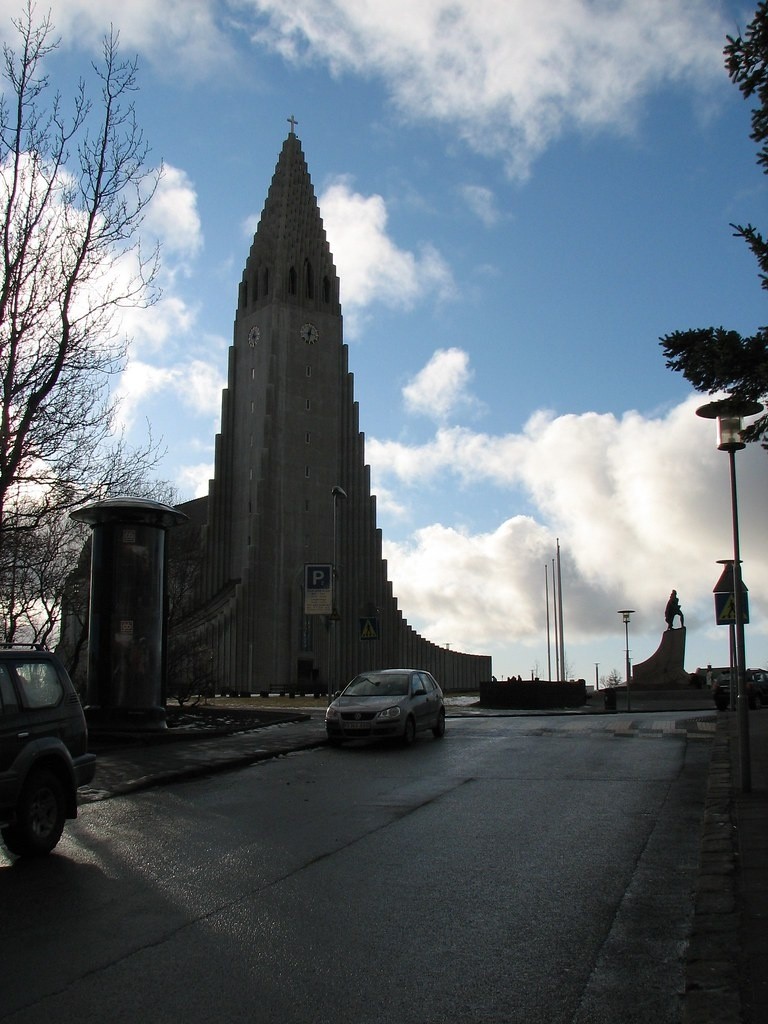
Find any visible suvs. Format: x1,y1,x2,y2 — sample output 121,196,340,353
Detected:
0,642,96,856
711,668,768,712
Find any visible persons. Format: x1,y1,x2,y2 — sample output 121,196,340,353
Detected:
665,590,686,630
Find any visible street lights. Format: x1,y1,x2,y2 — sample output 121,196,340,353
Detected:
331,486,347,697
696,399,764,795
594,663,600,690
617,610,635,685
628,658,633,676
715,560,743,712
531,670,534,681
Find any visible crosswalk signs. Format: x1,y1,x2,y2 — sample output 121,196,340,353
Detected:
359,617,379,640
715,593,749,625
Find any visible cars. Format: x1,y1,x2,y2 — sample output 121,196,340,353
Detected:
325,669,445,747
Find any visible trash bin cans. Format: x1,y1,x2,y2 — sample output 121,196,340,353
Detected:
603,688,617,710
